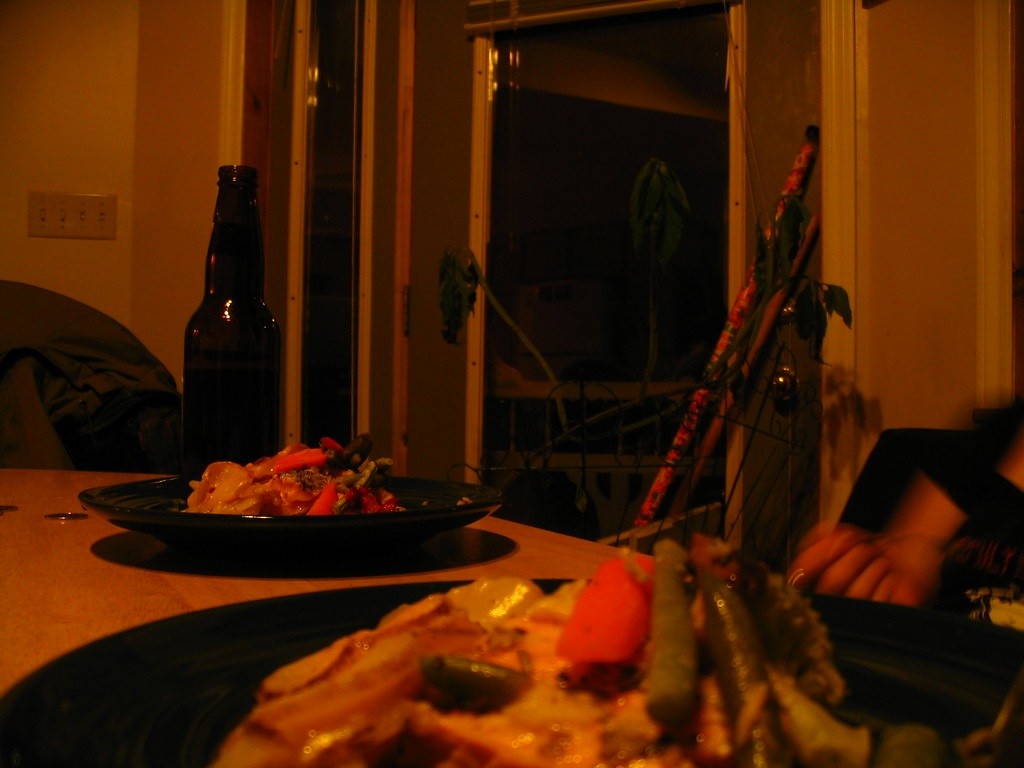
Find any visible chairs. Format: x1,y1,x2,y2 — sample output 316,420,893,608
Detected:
1,279,183,473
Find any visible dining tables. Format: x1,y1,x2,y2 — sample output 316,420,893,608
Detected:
0,468,1024,766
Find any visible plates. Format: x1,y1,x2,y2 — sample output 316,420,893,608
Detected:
0,578,1024,768
77,476,501,556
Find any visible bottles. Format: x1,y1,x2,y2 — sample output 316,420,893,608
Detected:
179,166,282,478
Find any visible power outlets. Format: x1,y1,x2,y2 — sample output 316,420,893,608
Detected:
28,193,119,239
